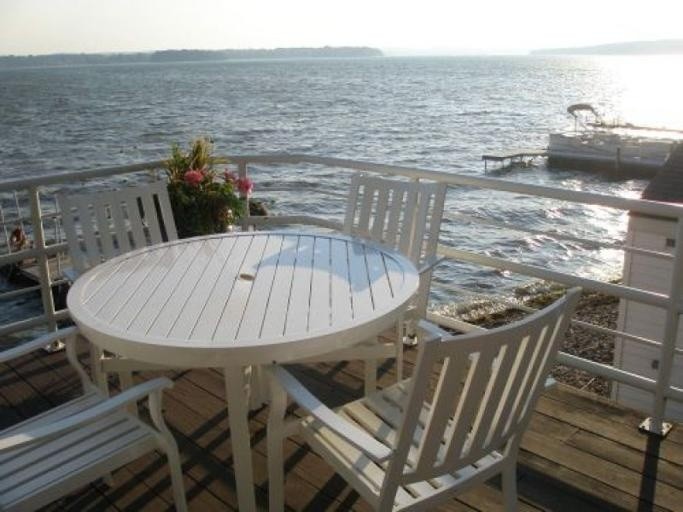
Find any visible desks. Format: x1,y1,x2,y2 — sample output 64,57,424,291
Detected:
66,230,420,511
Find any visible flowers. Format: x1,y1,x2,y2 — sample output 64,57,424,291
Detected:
165,136,253,233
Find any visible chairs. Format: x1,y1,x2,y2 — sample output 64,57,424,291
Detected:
342,173,448,394
0,325,188,511
58,181,264,424
268,287,582,511
